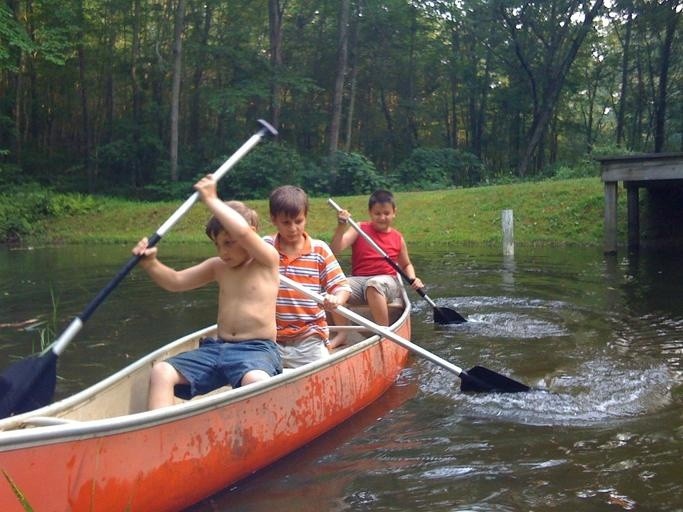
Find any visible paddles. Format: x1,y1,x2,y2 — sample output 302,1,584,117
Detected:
0,119,278,420
279,274,556,393
327,200,467,324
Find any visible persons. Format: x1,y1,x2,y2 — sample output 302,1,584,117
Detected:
260,183,353,374
128,173,284,412
326,188,425,350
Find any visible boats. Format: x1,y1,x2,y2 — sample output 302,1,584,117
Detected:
0,258,413,512
